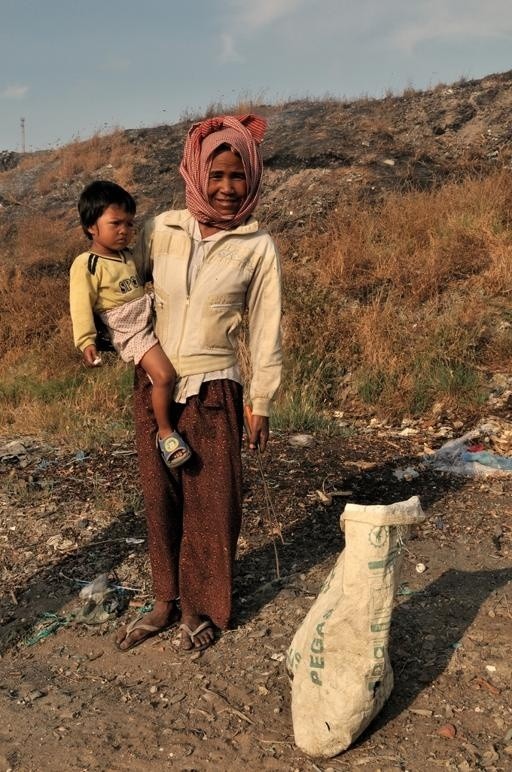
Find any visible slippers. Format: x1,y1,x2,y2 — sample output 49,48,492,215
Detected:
178,621,214,650
112,617,180,650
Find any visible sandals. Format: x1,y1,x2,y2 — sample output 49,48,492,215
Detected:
155,429,193,470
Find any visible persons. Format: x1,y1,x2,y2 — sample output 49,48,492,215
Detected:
68,180,193,467
92,112,285,653
